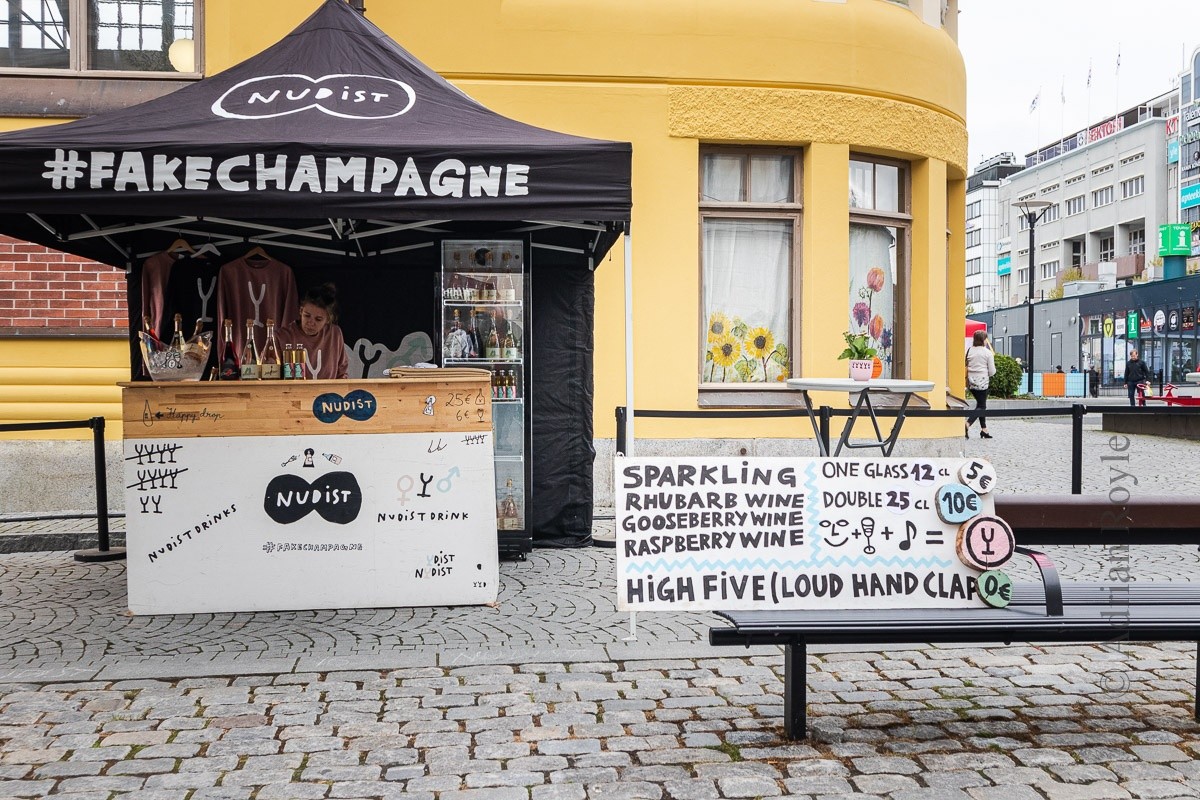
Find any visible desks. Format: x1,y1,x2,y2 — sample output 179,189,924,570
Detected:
786,377,934,457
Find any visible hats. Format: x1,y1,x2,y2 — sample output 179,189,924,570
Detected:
1056,365,1062,369
1071,365,1076,369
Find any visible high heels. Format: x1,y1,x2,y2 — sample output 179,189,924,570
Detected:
980,431,993,439
965,424,969,439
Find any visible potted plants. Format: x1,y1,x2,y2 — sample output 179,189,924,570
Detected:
838,330,878,381
1062,269,1108,298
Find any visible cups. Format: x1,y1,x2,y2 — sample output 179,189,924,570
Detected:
481,290,488,300
463,290,470,300
506,289,515,300
488,290,496,300
501,289,506,300
470,289,479,300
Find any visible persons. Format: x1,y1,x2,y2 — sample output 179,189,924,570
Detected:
273,286,349,379
965,330,996,438
1124,349,1151,406
1056,364,1097,398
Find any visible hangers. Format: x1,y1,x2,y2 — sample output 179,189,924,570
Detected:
190,232,221,258
167,228,195,254
244,239,269,260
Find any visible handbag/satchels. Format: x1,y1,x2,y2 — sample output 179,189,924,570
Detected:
965,366,968,387
1137,383,1153,396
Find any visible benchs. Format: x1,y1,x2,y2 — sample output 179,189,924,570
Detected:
708,495,1200,742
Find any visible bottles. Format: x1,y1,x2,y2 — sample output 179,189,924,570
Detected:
259,319,282,380
500,479,519,530
497,370,506,398
282,344,294,380
496,502,500,530
485,310,500,362
169,314,186,352
187,323,203,366
220,319,240,381
241,319,261,380
144,316,163,353
466,307,482,362
294,344,306,380
501,309,516,362
506,370,517,398
444,309,476,362
491,370,497,398
208,366,218,381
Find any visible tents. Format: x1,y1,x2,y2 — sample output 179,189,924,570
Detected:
0,0,640,641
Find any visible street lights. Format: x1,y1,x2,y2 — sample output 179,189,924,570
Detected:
1011,200,1054,400
